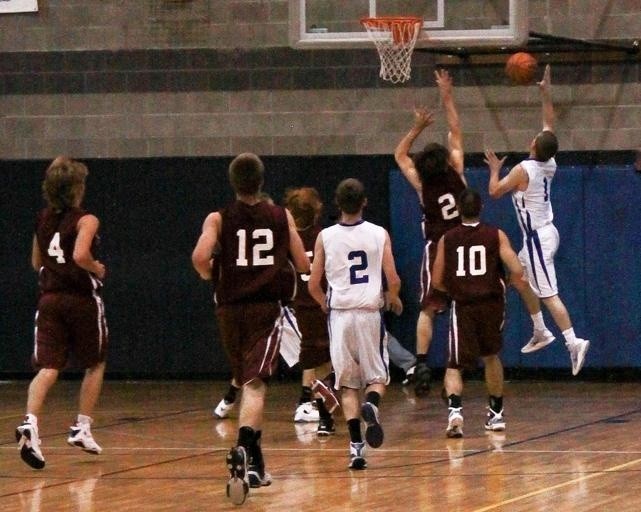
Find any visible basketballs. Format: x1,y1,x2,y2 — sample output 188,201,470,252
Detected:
509,51,536,80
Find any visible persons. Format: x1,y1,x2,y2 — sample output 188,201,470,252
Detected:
432,189,526,439
279,185,342,437
13,155,111,471
480,62,590,378
307,177,406,470
212,196,320,424
385,327,417,386
190,152,309,508
393,68,467,405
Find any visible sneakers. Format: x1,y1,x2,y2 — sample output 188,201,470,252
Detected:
484,408,505,430
447,407,464,437
16,423,45,468
67,423,102,455
401,364,431,397
294,379,340,436
521,329,555,354
226,446,272,504
348,402,383,469
214,399,234,417
568,339,590,375
